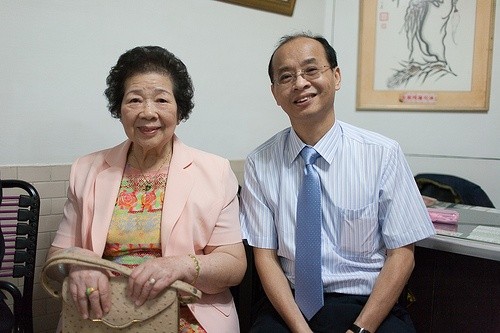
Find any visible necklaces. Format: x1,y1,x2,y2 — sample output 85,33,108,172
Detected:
134,150,171,190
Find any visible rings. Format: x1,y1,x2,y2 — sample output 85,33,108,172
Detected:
86,288,98,295
147,278,156,284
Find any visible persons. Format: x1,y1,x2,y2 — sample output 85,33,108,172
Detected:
240,32,437,333
45,45,248,333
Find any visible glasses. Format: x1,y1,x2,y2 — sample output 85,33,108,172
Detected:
270,65,331,85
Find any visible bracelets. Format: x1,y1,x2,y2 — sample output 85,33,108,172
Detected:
189,253,200,287
58,263,66,279
348,323,370,333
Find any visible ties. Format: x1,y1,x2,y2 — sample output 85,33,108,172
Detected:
294,147,324,319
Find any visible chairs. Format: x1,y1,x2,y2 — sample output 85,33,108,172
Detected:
413,173,497,210
0,177,41,333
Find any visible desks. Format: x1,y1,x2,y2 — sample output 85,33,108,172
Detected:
395,191,500,333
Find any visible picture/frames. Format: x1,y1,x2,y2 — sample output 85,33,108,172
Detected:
355,0,497,112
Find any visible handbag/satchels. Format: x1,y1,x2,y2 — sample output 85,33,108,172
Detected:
42,249,202,333
